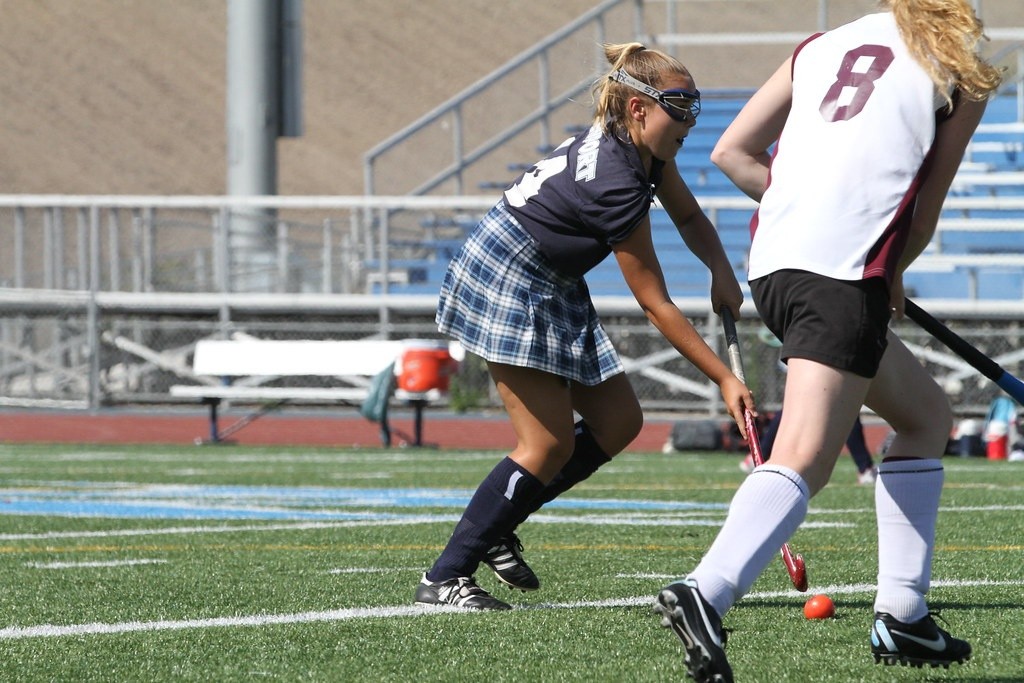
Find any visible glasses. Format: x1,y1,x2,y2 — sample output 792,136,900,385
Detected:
658,88,701,123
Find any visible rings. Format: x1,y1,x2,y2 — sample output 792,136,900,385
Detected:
891,307,896,312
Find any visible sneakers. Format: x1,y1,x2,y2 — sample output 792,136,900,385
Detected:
870,606,972,668
652,578,734,683
415,572,512,611
857,465,878,484
482,533,539,591
739,451,755,475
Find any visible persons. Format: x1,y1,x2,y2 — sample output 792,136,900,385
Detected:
656,0,1007,683
412,42,759,611
738,403,877,485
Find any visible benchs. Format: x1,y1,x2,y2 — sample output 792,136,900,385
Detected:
169,339,451,449
361,87,1023,300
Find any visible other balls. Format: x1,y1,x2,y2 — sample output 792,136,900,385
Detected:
804,594,834,620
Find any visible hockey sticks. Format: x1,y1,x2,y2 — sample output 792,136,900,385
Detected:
720,307,808,593
903,296,1024,405
